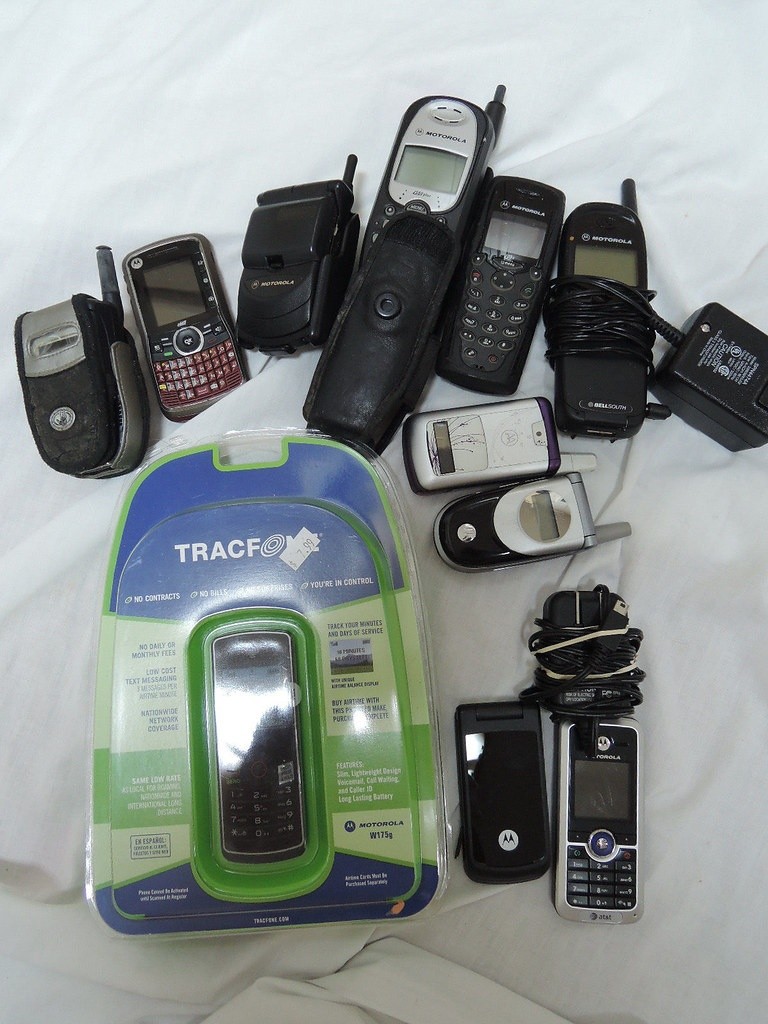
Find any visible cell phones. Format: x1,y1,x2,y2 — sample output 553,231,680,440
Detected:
16,82,649,925
